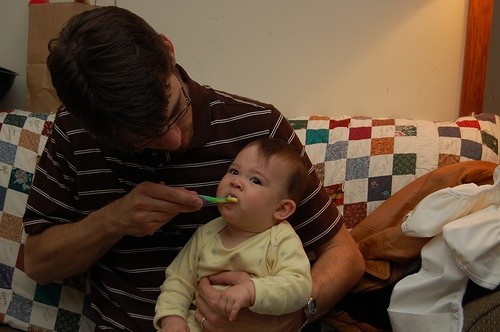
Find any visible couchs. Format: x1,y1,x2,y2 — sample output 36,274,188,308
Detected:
0,107,499,332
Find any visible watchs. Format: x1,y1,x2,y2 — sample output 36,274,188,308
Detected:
307,298,318,316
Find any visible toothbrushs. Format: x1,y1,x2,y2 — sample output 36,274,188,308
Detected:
194,194,239,205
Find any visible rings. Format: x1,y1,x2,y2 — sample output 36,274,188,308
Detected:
199,315,207,329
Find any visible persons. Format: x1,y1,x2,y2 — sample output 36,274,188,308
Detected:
21,5,366,331
152,136,312,332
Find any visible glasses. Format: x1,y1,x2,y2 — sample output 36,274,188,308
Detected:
137,70,192,153
